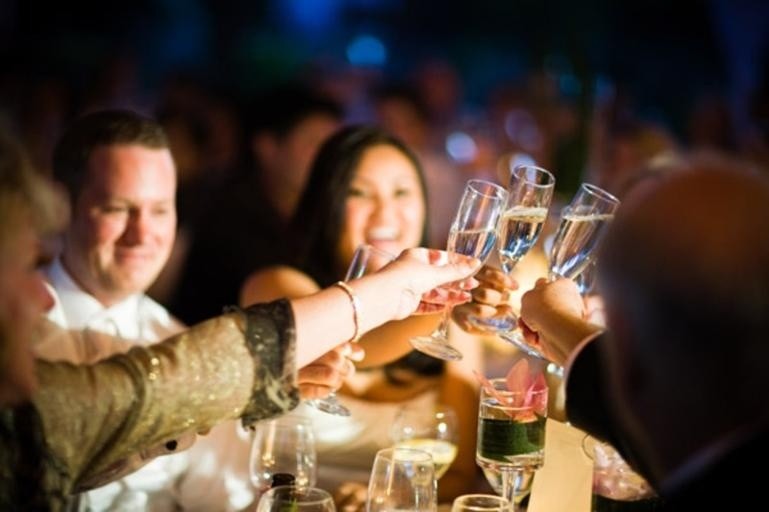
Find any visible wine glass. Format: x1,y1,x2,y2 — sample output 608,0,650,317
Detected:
306,244,396,419
251,419,337,512
409,177,510,361
502,179,620,356
363,404,459,511
454,370,546,509
466,163,554,340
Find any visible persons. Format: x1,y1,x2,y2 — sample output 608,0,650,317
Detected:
242,123,521,404
0,66,768,323
29,107,366,511
1,129,481,512
519,157,767,512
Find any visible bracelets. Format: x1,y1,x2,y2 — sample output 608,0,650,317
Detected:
336,280,361,343
